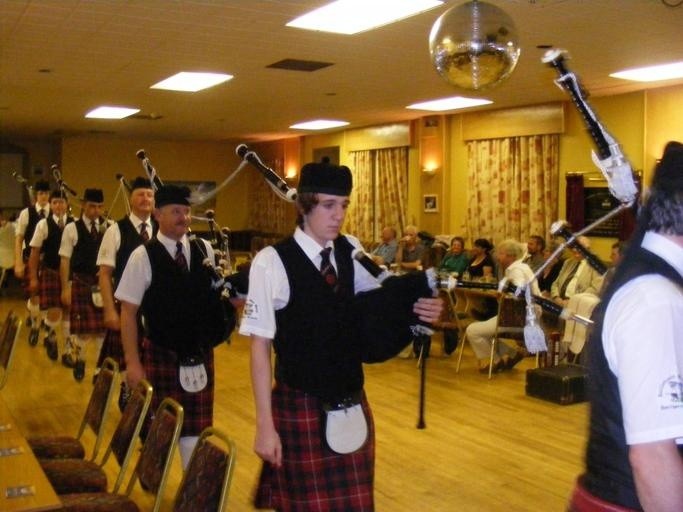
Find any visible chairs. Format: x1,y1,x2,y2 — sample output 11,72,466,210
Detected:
357,239,583,383
168,427,238,511
40,379,153,494
23,357,119,461
1,308,23,389
55,396,183,512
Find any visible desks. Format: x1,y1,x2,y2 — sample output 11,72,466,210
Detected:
2,397,65,511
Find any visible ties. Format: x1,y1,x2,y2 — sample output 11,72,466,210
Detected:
40,209,44,220
58,216,64,230
140,223,149,241
175,242,188,272
90,220,97,240
319,247,337,293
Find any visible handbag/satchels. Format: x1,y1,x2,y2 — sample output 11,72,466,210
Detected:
177,354,208,394
321,398,367,456
91,284,103,308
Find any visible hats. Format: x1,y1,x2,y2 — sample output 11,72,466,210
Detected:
131,177,152,191
298,157,352,196
154,185,191,207
85,189,103,202
34,179,49,191
48,190,68,203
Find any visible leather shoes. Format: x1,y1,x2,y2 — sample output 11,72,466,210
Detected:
26,316,85,381
119,382,131,413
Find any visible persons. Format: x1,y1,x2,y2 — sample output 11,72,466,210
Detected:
236,155,445,512
566,138,683,511
6,178,159,384
366,222,623,373
115,184,245,481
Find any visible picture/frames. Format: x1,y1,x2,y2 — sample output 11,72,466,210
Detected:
421,193,438,214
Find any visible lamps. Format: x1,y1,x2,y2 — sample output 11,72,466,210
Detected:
284,175,297,186
425,2,524,95
421,167,436,178
